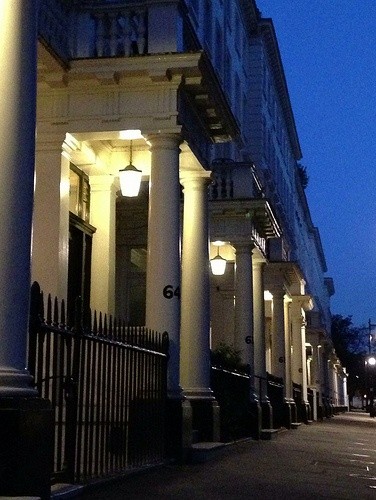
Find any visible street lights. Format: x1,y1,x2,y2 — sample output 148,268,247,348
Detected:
364,357,376,412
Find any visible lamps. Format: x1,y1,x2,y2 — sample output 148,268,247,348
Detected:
117,139,143,198
209,241,227,276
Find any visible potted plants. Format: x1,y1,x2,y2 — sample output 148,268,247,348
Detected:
212,337,241,370
